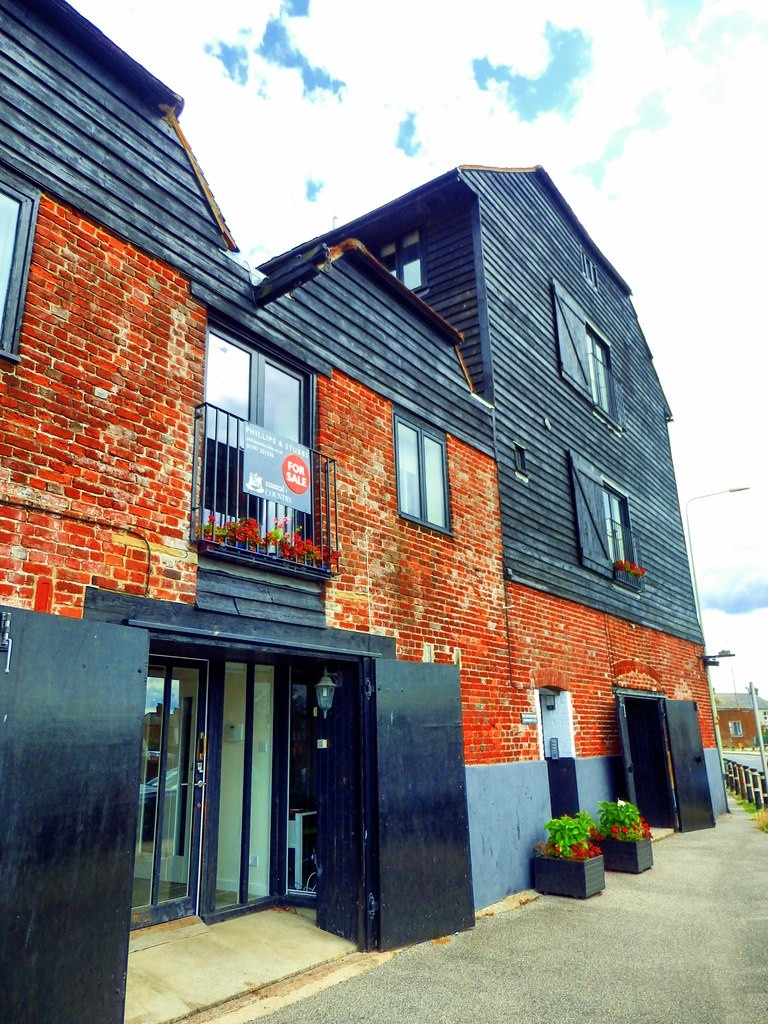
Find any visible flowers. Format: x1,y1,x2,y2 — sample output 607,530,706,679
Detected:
196,514,339,565
535,811,603,859
593,799,651,841
613,560,646,575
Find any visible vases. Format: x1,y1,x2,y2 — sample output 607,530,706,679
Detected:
599,836,654,873
204,537,331,577
533,855,605,897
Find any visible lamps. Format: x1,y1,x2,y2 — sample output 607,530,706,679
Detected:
546,695,555,709
314,665,337,718
